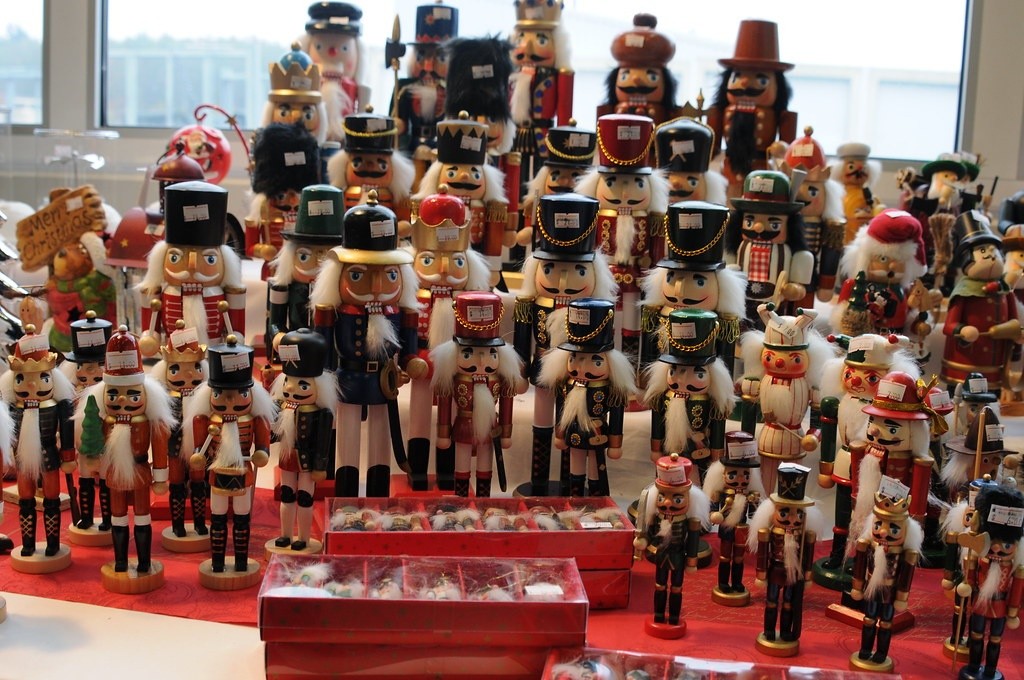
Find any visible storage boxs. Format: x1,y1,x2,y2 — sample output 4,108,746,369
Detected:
257,554,588,647
540,646,903,680
324,496,636,611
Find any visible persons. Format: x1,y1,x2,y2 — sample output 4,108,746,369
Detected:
0,1,1024,680
